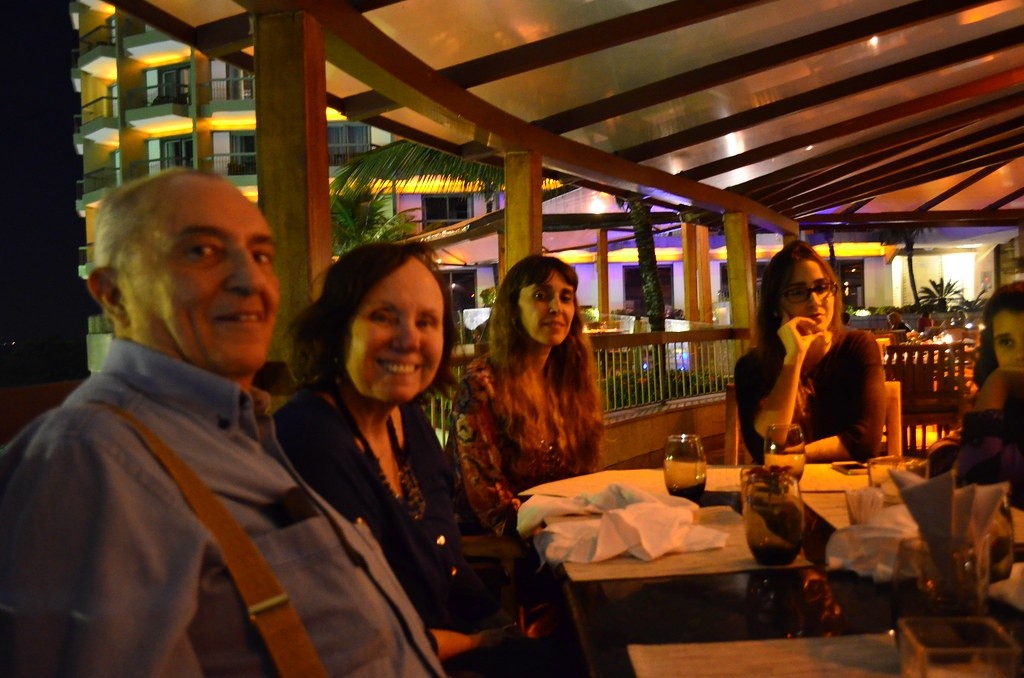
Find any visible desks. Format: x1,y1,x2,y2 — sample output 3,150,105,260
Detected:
559,464,1024,678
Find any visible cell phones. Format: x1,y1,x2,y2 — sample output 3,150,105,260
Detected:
831,460,869,475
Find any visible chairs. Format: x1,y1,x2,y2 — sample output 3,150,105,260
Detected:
457,534,524,618
723,381,903,465
884,342,964,455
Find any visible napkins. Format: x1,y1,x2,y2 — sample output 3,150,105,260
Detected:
825,468,1011,585
532,500,730,577
515,481,700,543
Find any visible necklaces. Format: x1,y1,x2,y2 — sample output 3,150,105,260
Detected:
335,388,426,521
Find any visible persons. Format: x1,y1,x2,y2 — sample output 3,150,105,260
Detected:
917,312,933,332
840,312,851,327
0,168,448,678
441,253,604,605
887,312,911,331
928,280,1024,512
268,245,561,678
952,310,967,326
734,237,885,464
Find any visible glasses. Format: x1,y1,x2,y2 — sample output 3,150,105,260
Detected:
776,278,838,303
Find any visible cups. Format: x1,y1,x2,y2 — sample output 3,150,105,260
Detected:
988,495,1016,582
890,539,983,645
742,468,804,566
763,422,806,482
664,434,707,504
896,615,1021,678
870,454,930,503
845,486,884,525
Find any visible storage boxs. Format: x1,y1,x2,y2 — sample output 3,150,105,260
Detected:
894,615,1021,678
